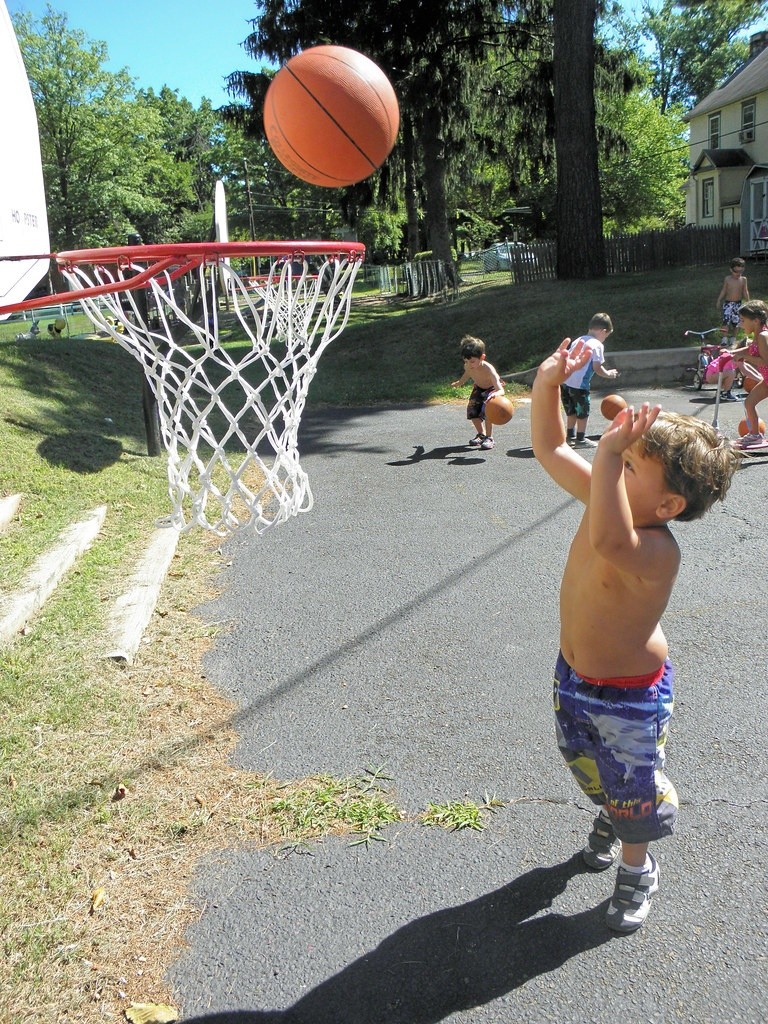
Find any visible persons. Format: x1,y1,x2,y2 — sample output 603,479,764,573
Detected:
451,335,505,450
529,335,732,932
559,313,619,449
705,257,768,445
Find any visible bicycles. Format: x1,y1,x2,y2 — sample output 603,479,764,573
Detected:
685,323,745,392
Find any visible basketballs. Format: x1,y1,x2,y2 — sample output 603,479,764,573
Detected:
738,417,766,438
484,396,514,425
601,394,628,421
743,375,760,393
263,42,401,190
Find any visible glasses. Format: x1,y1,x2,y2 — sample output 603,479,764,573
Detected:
732,268,744,274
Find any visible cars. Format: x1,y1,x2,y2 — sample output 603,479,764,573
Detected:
484,241,541,272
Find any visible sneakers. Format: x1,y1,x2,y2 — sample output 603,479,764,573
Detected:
735,433,763,445
729,336,736,346
469,433,486,446
604,850,661,932
566,436,577,446
719,390,738,400
721,336,728,346
583,809,622,869
574,438,598,448
481,436,494,450
